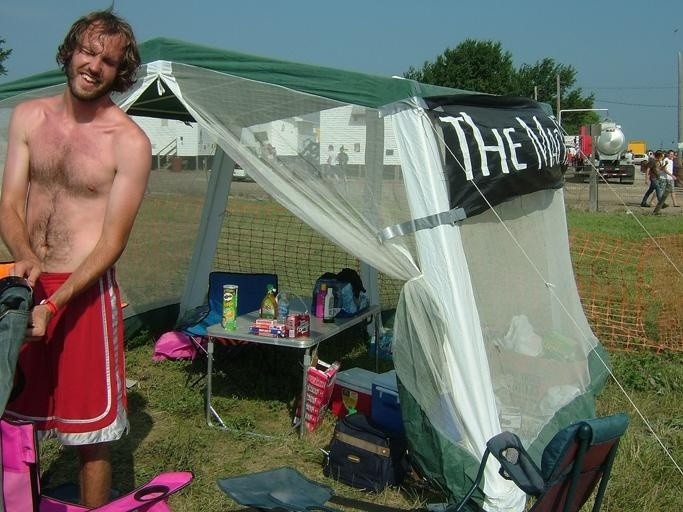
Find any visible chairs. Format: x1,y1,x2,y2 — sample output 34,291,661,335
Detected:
176,272,279,389
457,413,629,512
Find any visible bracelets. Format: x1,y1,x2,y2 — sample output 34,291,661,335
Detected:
38,298,57,315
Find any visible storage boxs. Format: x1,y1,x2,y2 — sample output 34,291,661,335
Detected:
332,366,407,445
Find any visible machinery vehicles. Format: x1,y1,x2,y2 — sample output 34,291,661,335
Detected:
571,111,635,185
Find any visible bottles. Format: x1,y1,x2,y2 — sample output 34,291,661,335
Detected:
278,292,290,324
316,283,334,318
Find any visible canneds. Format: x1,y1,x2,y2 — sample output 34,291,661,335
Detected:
285,313,310,339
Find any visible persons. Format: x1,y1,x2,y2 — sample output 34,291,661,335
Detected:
640,149,669,209
336,145,351,182
0,0,154,508
324,144,336,184
259,140,276,167
626,150,678,185
649,150,681,207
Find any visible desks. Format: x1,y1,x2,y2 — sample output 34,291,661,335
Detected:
206,296,383,443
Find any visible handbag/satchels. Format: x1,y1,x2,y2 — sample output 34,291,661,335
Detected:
310,267,369,318
321,410,405,493
